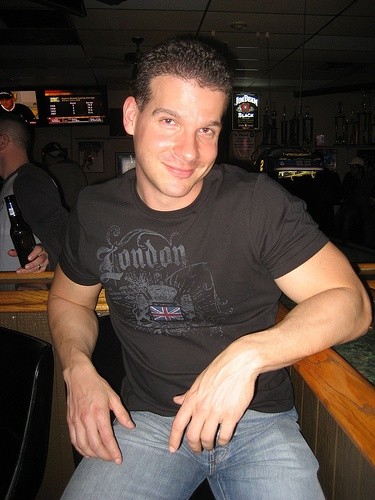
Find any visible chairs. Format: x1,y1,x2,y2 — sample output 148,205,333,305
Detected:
0,326,54,500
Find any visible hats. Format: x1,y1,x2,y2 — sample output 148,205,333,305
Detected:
0,91,14,100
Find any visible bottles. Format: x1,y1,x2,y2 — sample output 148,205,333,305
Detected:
4,194,36,269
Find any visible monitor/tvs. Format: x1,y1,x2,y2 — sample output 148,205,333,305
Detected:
0,87,48,126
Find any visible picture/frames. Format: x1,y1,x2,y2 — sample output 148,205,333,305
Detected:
231,91,260,131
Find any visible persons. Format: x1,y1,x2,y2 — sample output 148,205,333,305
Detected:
42,142,88,213
0,117,68,275
47,35,372,500
0,90,36,119
301,152,369,243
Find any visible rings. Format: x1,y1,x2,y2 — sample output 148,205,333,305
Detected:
38,264,41,270
